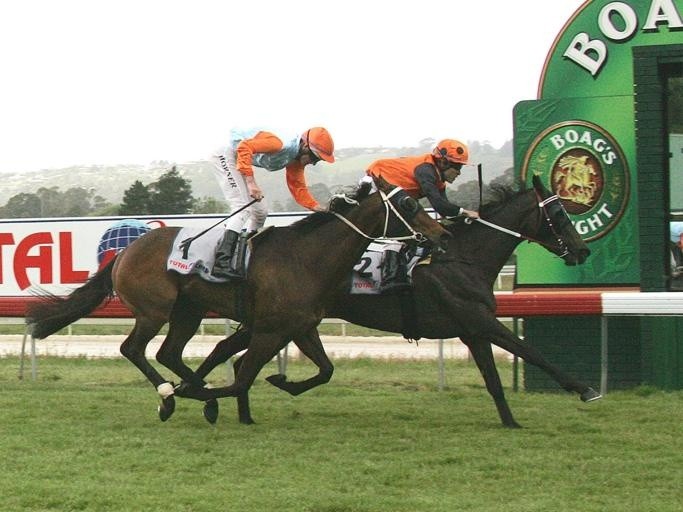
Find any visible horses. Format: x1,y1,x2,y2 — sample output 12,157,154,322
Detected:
190,166,606,430
23,167,454,427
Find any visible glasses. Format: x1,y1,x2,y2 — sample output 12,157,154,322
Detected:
451,164,462,171
309,153,321,163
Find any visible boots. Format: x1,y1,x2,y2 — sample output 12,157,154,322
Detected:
379,249,411,295
211,229,244,281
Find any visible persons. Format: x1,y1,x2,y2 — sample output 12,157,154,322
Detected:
204,121,342,284
357,139,480,301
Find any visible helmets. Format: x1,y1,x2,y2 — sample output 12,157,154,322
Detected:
299,127,335,164
431,139,472,166
